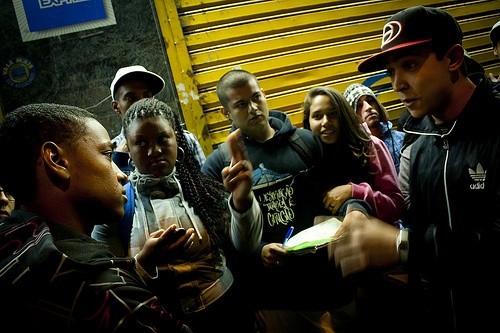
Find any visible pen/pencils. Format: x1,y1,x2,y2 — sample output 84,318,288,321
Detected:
276,227,293,264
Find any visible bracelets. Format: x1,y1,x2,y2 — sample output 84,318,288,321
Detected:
395,228,409,261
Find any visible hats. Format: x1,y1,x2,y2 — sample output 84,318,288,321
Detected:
342,83,389,124
357,5,464,73
489,20,500,47
109,64,166,101
463,48,485,74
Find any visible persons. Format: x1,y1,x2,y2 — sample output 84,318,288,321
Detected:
0,22,500,333
325,4,500,333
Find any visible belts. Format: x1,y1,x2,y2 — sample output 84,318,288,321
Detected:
180,265,234,315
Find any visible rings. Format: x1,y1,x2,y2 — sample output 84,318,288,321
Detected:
329,203,335,208
